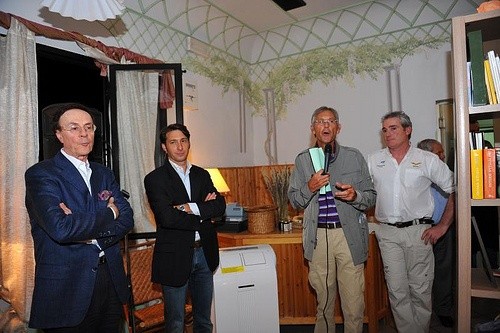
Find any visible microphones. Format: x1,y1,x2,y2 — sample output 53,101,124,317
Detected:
323,143,332,174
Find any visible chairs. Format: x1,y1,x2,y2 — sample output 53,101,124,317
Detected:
118,240,195,329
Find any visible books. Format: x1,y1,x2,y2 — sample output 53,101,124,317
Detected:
468,130,497,199
466,29,500,105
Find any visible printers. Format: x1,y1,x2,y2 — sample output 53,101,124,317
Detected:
210,202,249,233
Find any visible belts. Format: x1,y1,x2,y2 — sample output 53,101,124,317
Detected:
378,218,434,228
194,241,202,248
99,256,106,265
316,221,342,230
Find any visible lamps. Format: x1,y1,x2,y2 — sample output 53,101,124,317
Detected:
204,168,231,194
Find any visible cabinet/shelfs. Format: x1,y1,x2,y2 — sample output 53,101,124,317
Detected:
450,10,500,333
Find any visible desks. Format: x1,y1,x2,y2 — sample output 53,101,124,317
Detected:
216,226,389,333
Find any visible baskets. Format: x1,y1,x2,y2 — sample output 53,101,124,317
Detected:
243,203,279,234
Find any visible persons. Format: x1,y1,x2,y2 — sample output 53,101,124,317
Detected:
23,109,134,333
364,110,456,333
415,139,457,333
447,119,497,269
143,123,227,333
287,105,377,333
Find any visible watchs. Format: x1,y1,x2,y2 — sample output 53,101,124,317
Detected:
179,204,185,211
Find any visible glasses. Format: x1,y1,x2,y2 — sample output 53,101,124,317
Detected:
61,125,97,134
311,120,338,125
